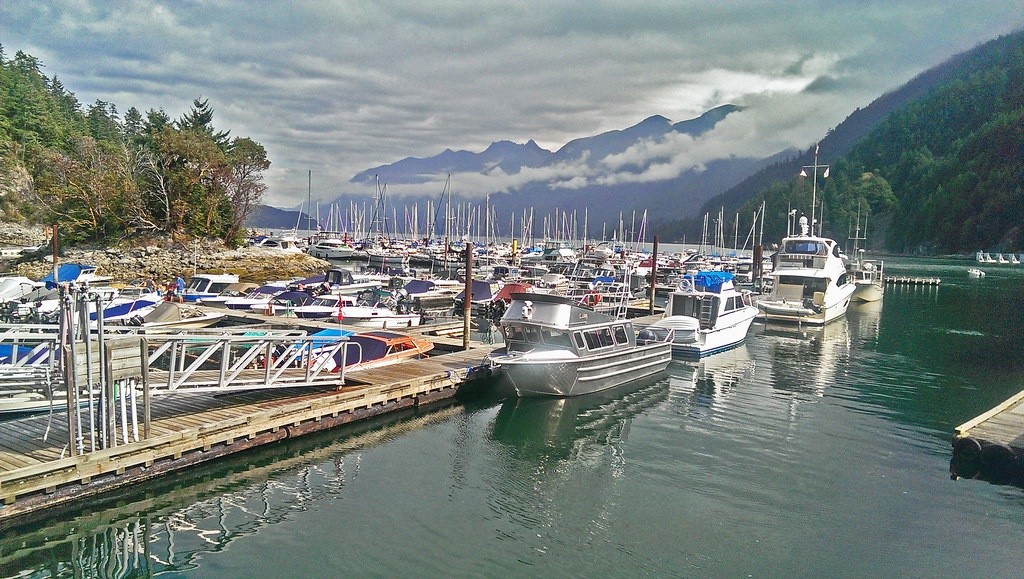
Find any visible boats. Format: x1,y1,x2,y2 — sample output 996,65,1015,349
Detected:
484,253,673,400
0,262,435,414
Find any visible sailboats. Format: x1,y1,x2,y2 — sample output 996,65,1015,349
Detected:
247,141,884,363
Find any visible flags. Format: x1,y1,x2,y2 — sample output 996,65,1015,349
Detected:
800,169,808,179
823,167,829,179
338,294,344,322
815,141,819,156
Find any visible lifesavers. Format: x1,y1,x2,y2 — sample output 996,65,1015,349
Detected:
678,278,692,292
582,290,600,308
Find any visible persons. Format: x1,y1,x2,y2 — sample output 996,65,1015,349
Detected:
166,273,187,303
